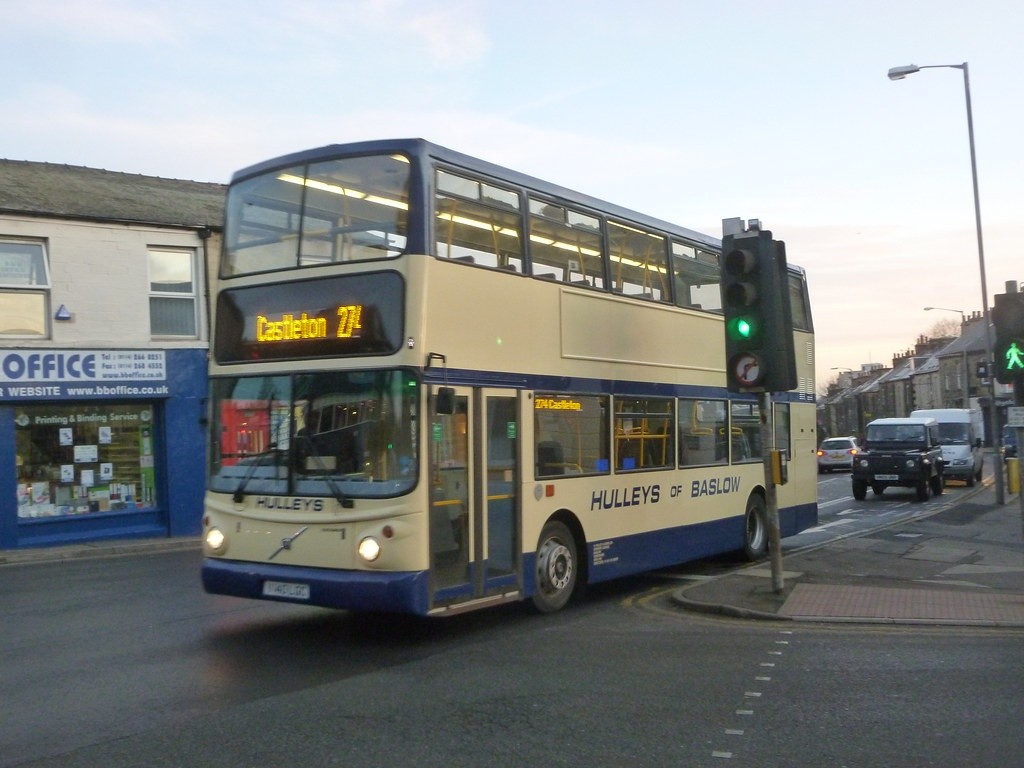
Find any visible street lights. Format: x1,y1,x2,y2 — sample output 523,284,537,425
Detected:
831,365,853,385
884,61,1008,512
921,302,974,410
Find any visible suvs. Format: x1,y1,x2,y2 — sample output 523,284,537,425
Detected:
850,417,942,502
818,436,862,473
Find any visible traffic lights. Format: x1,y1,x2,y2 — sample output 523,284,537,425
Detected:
720,235,772,392
994,294,1023,384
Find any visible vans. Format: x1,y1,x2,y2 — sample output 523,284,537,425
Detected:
909,408,985,488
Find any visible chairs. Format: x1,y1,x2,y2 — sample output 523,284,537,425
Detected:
615,426,752,467
455,256,723,313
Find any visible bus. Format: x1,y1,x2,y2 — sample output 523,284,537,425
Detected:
199,137,820,626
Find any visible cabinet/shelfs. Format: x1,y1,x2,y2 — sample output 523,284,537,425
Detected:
101,433,141,493
